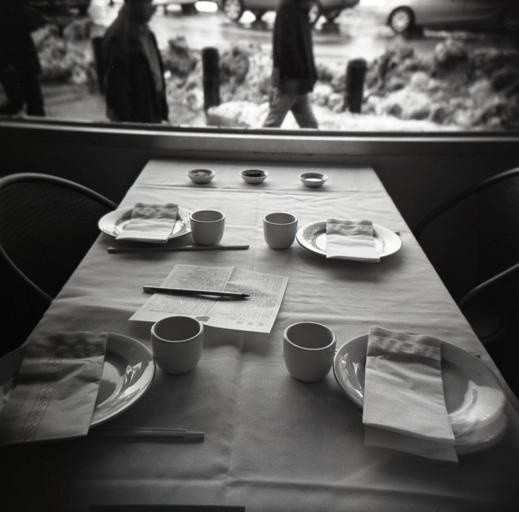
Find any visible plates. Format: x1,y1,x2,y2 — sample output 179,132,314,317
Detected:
295,216,404,262
96,204,194,240
1,333,153,431
187,168,330,188
333,331,508,437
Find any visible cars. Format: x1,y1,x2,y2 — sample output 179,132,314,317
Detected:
371,0,518,34
105,0,359,33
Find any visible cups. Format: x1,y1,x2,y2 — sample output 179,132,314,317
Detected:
150,315,203,376
283,322,336,383
191,209,226,245
264,213,297,249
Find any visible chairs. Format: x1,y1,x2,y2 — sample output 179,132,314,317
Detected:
414,168,519,377
0,172,116,305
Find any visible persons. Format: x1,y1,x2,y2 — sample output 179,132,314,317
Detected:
0,0,45,115
263,1,320,127
100,0,169,122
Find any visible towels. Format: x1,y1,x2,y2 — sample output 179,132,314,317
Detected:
117,199,178,246
324,216,378,261
0,331,110,446
359,325,460,464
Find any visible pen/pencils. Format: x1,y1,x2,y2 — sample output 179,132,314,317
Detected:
143,286,249,298
